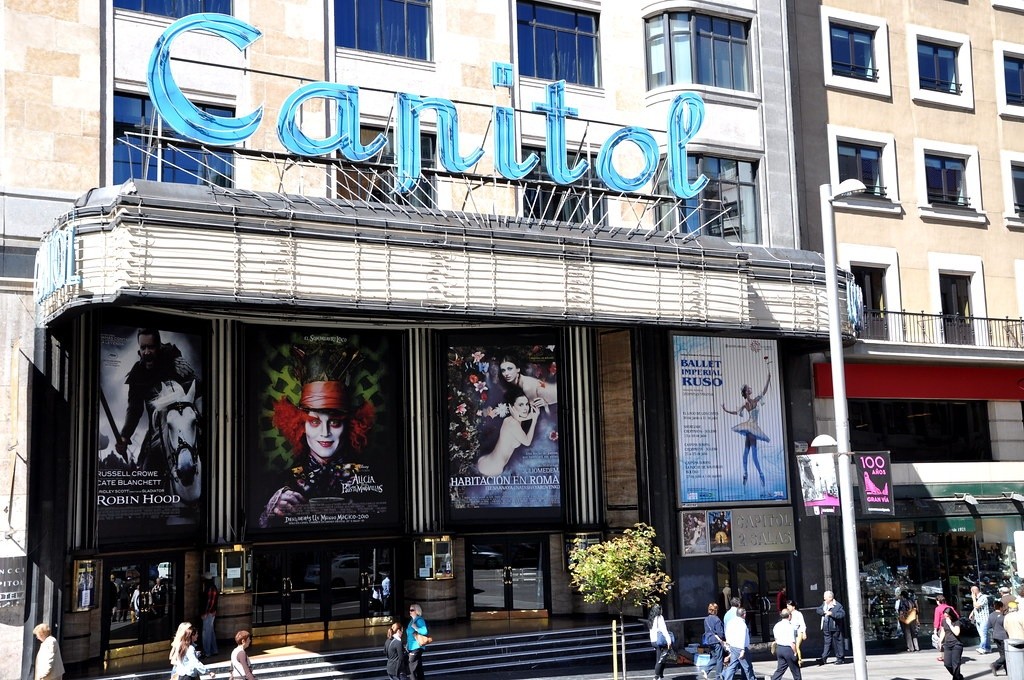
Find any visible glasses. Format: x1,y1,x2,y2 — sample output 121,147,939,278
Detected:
409,609,415,612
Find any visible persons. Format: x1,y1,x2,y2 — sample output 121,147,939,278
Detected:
370,572,391,616
255,340,385,527
169,622,216,680
405,604,428,680
229,630,259,680
475,354,559,477
721,371,771,486
871,580,904,638
110,573,167,624
770,609,803,680
815,590,846,665
648,604,672,680
786,601,807,667
384,622,409,680
900,579,1024,680
114,326,203,487
198,572,219,657
723,597,760,680
701,603,727,680
777,584,786,615
32,623,65,680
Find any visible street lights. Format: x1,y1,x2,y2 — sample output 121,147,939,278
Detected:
810,179,870,679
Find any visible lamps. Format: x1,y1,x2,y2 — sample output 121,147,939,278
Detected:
1011,493,1024,501
964,492,978,505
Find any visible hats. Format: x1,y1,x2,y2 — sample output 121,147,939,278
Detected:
201,571,213,579
287,334,367,415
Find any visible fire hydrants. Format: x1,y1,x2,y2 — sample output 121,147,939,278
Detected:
130,612,135,623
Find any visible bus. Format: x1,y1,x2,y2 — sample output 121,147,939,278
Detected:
747,557,785,594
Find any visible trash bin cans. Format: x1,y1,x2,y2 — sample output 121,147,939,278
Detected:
1004,639,1024,680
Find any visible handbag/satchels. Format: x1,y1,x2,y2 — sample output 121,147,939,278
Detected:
658,646,678,664
899,606,918,625
932,635,942,651
413,631,433,646
657,631,676,647
771,642,778,655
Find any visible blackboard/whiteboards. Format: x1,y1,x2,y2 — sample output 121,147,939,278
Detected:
0,555,27,627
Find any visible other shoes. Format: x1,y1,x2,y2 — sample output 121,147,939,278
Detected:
976,647,985,654
660,677,672,680
701,669,708,680
816,659,825,664
834,659,846,665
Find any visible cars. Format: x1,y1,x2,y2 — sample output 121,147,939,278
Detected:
862,595,897,619
921,578,944,605
441,544,502,569
305,554,358,595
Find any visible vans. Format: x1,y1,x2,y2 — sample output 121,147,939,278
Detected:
716,557,758,594
